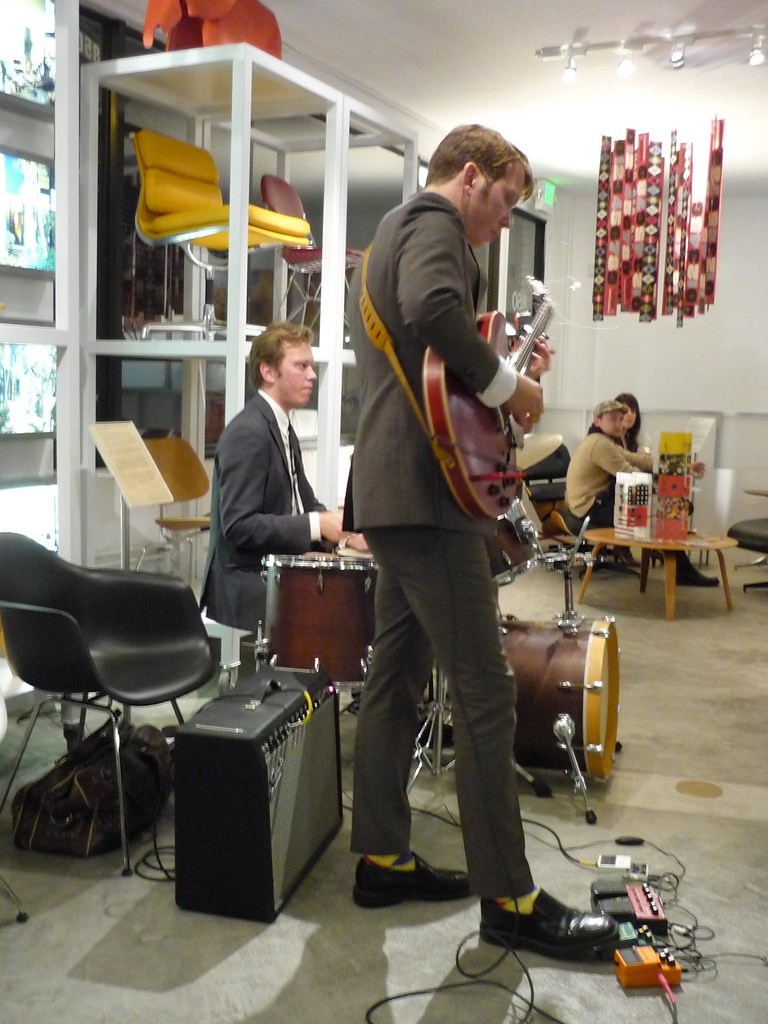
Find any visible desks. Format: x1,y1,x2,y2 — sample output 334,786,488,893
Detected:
577,528,739,621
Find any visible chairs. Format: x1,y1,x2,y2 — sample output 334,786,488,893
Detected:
727,518,768,593
261,168,367,341
136,437,214,586
514,434,642,579
1,532,216,874
120,127,310,352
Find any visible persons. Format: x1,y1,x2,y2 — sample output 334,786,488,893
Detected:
341,125,620,959
566,393,720,587
197,321,454,750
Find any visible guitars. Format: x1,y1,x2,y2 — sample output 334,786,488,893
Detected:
424,294,555,523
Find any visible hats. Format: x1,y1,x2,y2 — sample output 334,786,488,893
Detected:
593,400,628,417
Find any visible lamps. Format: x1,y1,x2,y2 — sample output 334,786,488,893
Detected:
560,44,586,80
747,31,767,66
615,36,644,72
669,37,694,68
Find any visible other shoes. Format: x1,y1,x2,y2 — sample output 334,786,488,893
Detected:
413,716,453,748
676,565,719,587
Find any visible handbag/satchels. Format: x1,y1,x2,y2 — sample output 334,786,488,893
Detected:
10,709,173,858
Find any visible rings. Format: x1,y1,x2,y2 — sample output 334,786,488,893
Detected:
532,416,539,422
526,412,531,417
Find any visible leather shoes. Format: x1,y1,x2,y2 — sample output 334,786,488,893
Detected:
479,885,618,958
352,851,473,908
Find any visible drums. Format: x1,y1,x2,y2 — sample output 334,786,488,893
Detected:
485,498,546,586
443,613,620,783
255,554,379,690
